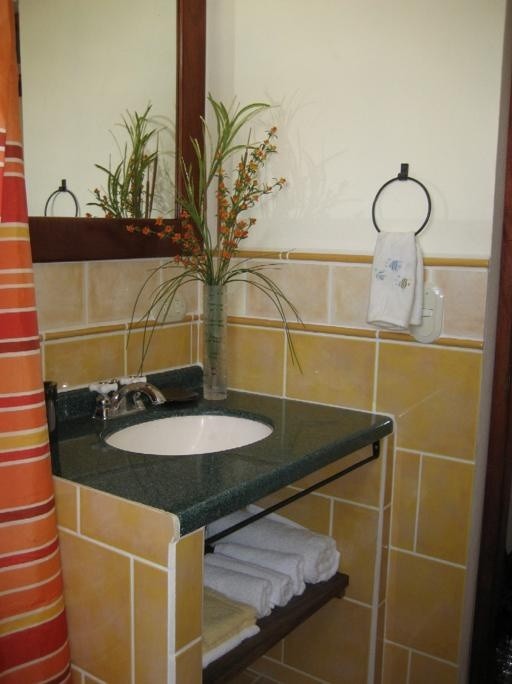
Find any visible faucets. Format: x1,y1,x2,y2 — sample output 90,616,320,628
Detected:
110,382,168,419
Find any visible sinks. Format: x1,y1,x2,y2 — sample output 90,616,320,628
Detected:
100,407,275,457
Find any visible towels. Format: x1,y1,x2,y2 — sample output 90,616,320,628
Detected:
367,231,423,331
206,510,341,584
213,542,306,596
204,553,294,607
202,586,257,652
202,565,273,619
202,625,260,670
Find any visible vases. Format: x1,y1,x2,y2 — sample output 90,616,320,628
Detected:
201,284,229,402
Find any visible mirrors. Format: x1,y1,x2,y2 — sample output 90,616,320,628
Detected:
15,0,206,265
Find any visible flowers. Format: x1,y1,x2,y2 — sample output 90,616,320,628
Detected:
84,103,175,220
119,95,307,377
